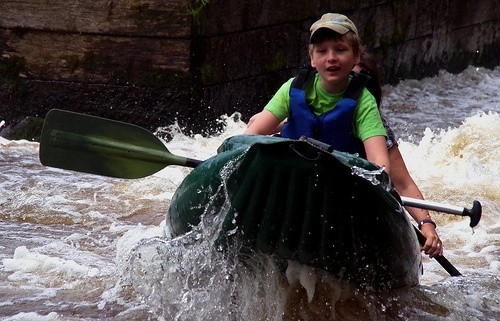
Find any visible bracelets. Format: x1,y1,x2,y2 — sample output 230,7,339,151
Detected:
417,219,438,230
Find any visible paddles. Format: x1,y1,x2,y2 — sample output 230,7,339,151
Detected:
39,109,482,228
299,136,464,278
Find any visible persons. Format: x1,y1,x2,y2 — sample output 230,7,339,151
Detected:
245,45,445,258
246,13,389,174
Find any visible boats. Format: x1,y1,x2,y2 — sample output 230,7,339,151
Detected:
165,135,421,289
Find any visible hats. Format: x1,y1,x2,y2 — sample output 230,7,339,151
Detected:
310,13,358,42
